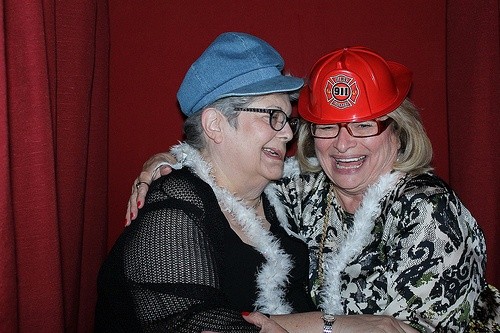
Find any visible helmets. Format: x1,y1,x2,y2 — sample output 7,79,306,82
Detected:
297,46,411,124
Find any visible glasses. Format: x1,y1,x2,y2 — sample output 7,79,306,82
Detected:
310,117,393,139
234,107,300,135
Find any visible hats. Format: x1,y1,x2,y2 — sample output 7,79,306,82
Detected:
177,31,304,119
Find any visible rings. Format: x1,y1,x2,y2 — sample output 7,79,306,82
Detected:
136,182,150,189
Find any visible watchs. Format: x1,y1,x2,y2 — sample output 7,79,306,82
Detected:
320,314,336,333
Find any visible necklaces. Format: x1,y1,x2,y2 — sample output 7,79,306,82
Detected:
317,182,332,286
250,196,261,211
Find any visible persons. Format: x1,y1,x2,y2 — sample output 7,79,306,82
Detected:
124,46,500,333
94,32,421,333
